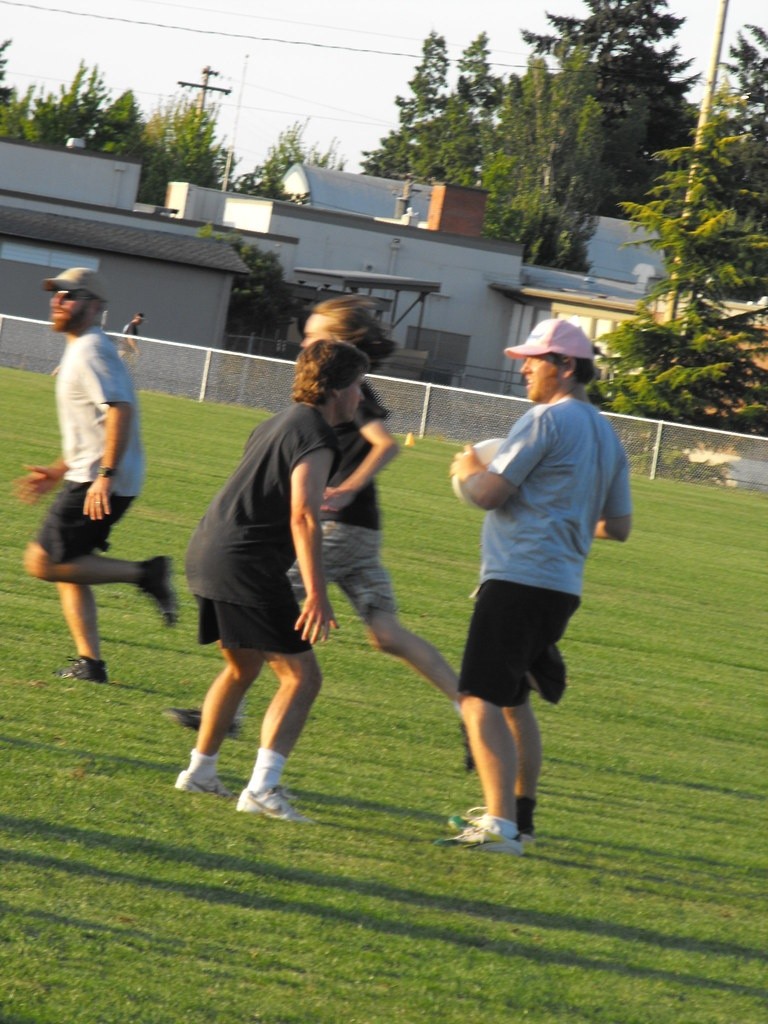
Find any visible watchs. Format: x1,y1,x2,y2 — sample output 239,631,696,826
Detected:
96,466,118,478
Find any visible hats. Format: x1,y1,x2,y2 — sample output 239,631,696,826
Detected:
504,319,595,359
43,266,108,302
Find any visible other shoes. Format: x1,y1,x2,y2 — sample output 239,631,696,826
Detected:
458,721,477,777
141,554,180,627
153,704,248,743
53,653,111,685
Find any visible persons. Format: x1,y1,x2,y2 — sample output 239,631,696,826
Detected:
117,313,143,389
12,267,174,682
432,319,633,856
161,294,475,769
175,340,368,826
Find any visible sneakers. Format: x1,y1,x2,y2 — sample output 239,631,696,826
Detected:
431,817,525,858
175,769,242,798
448,814,532,845
236,784,313,822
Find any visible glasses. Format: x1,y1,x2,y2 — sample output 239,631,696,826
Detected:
52,286,97,301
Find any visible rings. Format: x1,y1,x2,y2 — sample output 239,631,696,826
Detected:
94,502,101,504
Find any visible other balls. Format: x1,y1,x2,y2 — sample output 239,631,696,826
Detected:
452,439,510,500
